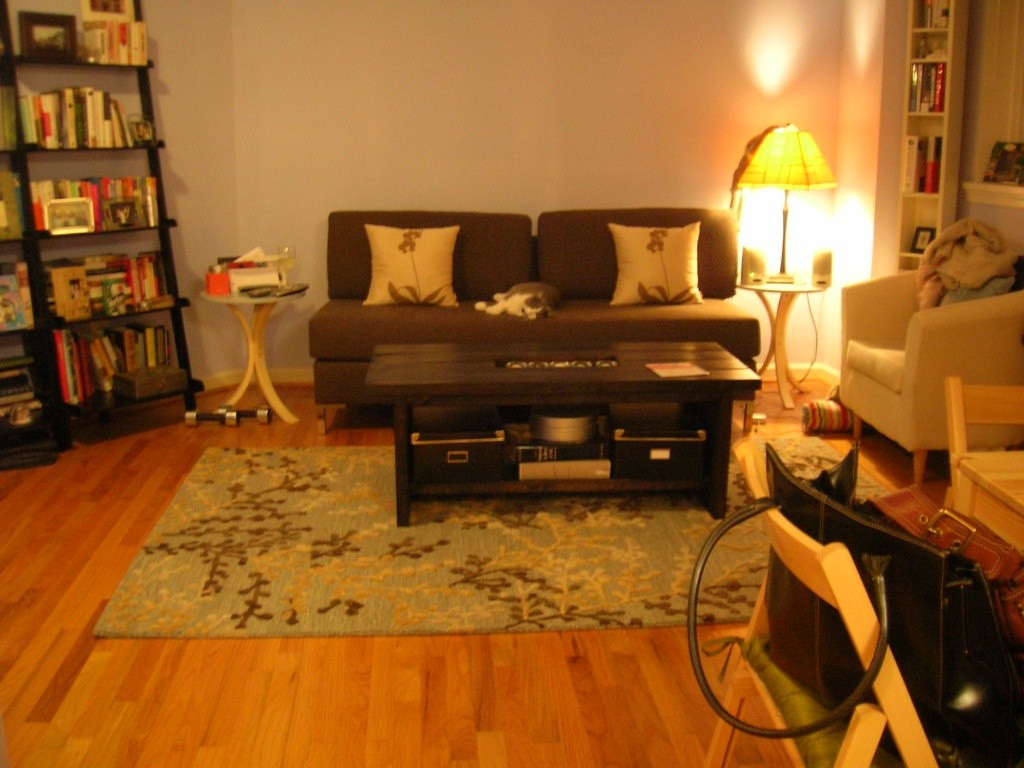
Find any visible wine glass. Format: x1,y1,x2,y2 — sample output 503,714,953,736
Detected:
277,246,296,290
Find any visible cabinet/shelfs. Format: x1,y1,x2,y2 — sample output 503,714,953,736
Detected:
895,0,969,272
1,0,204,471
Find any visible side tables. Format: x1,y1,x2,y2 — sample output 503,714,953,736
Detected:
199,282,306,424
736,274,827,410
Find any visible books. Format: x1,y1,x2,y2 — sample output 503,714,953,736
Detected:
0,320,171,417
0,168,160,241
908,62,945,112
902,135,944,193
920,0,950,28
0,250,173,334
0,0,148,66
0,81,153,152
645,361,709,378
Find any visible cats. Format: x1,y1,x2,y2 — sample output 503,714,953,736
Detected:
475,282,561,321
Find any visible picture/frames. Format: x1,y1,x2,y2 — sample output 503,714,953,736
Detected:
18,11,76,63
910,227,935,254
98,195,148,231
42,197,95,237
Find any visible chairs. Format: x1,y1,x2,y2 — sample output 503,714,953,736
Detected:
939,377,1023,508
703,455,942,768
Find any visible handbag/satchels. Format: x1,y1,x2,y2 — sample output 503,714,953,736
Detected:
868,484,1024,642
684,442,1024,768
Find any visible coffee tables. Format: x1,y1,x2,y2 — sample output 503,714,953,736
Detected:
362,341,763,527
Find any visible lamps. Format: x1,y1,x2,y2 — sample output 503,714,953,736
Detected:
736,124,837,284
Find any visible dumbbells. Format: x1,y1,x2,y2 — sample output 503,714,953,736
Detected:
183,404,273,428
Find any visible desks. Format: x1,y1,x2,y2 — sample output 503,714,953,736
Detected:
950,453,1024,556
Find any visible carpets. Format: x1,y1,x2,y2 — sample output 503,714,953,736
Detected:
89,432,890,637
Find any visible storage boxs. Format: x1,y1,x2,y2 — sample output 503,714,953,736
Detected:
411,406,506,483
613,428,707,480
517,415,612,480
204,270,231,297
112,364,187,401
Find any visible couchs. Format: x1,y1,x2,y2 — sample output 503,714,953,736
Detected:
308,206,760,434
839,268,1023,485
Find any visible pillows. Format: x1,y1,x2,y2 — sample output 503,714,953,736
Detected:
607,220,703,304
938,276,1014,306
802,400,854,433
363,223,460,307
